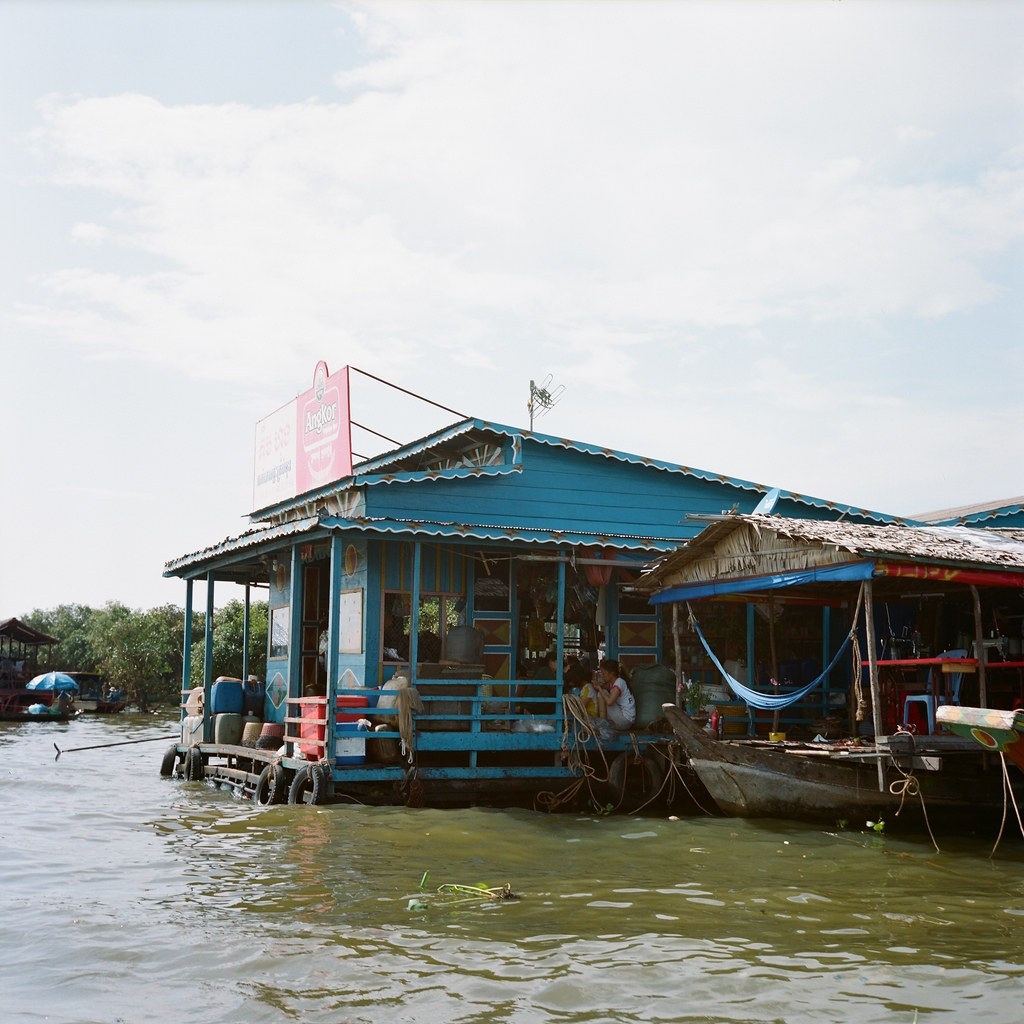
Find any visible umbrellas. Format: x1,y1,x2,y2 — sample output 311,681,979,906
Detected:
25,670,79,699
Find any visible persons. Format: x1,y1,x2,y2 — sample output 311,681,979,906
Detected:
58,690,83,715
0,650,25,679
102,681,117,702
317,615,329,677
520,651,636,732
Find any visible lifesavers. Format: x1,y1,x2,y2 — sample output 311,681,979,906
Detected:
160,748,176,777
254,764,285,806
184,747,203,781
288,764,326,808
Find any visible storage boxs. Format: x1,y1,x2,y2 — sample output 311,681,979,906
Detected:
299,695,369,765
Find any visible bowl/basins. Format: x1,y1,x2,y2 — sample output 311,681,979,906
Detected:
768,732,786,741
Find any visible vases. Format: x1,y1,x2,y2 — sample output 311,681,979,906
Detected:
716,705,747,735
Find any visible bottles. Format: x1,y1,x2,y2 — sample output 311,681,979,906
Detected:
242,710,260,723
706,710,725,737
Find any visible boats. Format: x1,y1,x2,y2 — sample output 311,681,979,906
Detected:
0,671,129,724
660,701,1024,838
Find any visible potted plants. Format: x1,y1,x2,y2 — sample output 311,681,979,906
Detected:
681,681,712,728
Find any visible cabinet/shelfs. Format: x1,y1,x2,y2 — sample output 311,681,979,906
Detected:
413,664,486,731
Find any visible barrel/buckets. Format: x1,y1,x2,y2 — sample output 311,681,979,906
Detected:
182,677,265,744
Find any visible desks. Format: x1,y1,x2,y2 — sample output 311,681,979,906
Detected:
860,658,979,733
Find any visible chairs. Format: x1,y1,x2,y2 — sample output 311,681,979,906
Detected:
903,648,968,736
